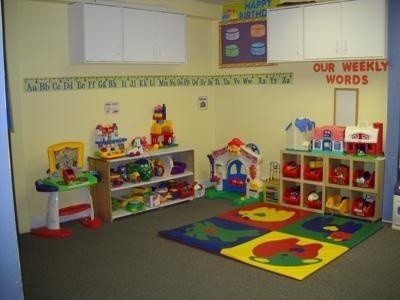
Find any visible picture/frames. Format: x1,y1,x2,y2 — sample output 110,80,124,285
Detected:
334,88,358,127
219,17,277,68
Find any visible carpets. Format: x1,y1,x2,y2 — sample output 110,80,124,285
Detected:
159,202,384,280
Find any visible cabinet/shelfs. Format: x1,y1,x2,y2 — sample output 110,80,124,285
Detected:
279,150,384,223
88,148,195,224
68,0,185,64
267,1,388,63
